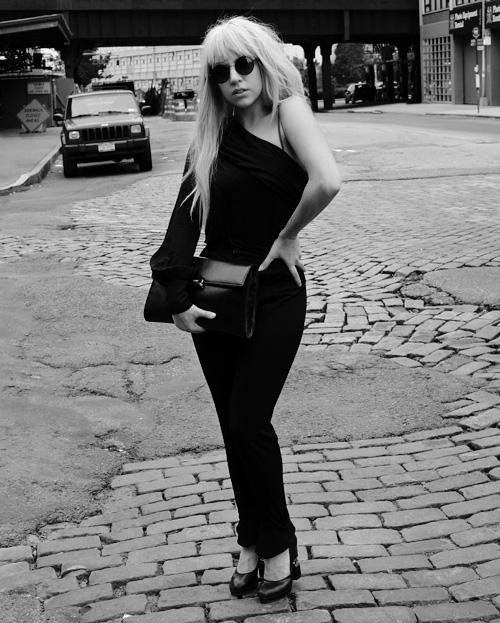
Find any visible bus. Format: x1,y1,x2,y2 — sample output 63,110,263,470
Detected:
92,80,136,96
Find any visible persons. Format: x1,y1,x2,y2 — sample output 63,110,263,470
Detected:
147,15,341,599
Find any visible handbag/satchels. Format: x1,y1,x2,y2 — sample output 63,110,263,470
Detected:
143,256,261,339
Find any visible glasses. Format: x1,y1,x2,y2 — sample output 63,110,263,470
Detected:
211,56,255,83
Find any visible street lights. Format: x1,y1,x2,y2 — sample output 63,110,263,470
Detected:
472,27,479,103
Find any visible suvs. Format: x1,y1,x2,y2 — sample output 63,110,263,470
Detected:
345,83,373,104
52,91,152,177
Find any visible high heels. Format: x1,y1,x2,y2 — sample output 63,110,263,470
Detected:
229,535,298,600
